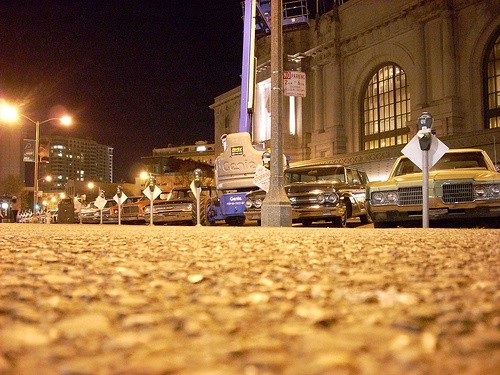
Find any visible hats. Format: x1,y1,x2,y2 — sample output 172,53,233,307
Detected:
12,196,17,199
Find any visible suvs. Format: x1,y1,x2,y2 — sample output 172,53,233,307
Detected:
110,196,151,222
365,149,500,228
284,163,373,228
144,187,193,223
78,199,117,223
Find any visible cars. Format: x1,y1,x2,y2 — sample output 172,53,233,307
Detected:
52,208,80,224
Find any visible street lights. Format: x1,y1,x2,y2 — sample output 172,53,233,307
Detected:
88,182,101,197
3,107,70,223
37,176,52,215
139,170,151,184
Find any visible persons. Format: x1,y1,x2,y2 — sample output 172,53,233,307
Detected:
11,195,20,223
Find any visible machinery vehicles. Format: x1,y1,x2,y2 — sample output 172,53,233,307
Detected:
192,0,311,226
